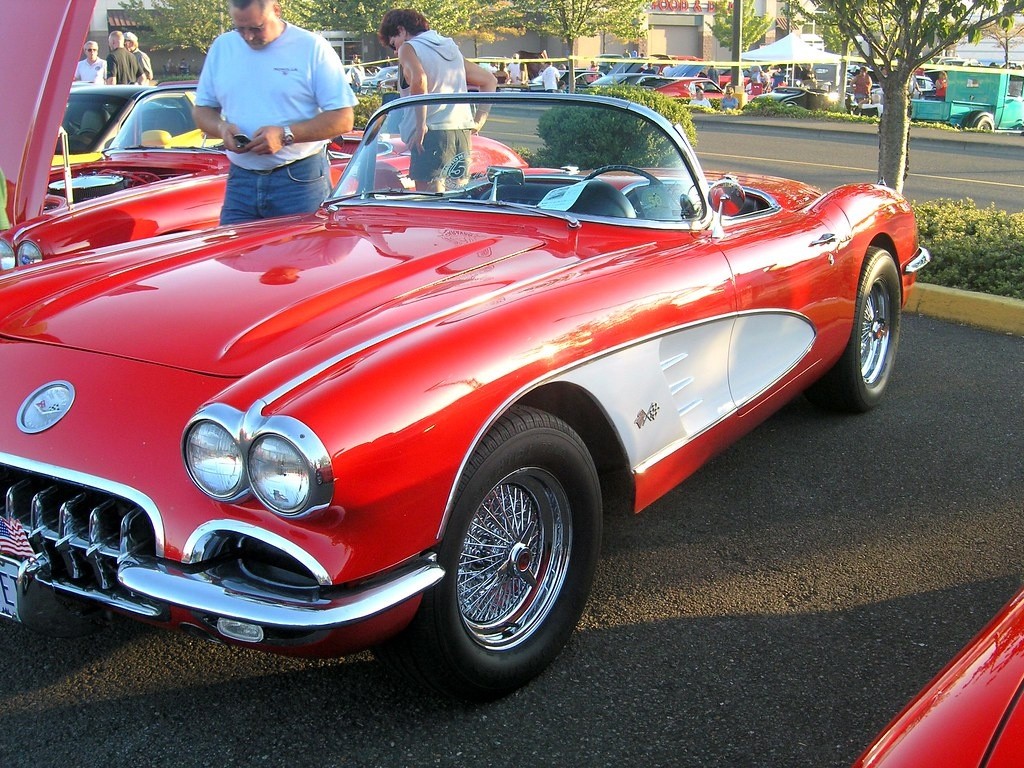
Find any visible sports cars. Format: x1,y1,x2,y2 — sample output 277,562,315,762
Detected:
0,0,885,279
0,91,931,706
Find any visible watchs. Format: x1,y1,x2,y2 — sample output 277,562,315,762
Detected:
283,125,295,145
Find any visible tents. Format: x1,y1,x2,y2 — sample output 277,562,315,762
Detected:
743,33,842,63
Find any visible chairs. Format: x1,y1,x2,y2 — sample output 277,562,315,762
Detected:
80,109,111,132
479,183,571,206
626,183,759,219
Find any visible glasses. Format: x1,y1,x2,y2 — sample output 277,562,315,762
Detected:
85,49,98,52
232,23,264,32
389,29,400,50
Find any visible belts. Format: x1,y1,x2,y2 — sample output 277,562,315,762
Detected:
249,160,300,175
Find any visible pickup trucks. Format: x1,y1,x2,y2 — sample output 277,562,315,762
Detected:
908,67,1024,134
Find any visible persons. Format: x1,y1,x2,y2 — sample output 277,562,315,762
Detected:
586,61,598,83
689,90,712,107
489,53,524,84
721,87,739,111
935,72,947,102
913,75,922,95
351,59,363,95
382,56,394,67
193,0,361,225
166,57,188,72
75,30,154,84
542,62,569,92
707,66,720,86
850,67,872,104
378,8,498,192
623,48,637,57
643,63,656,74
750,64,815,87
